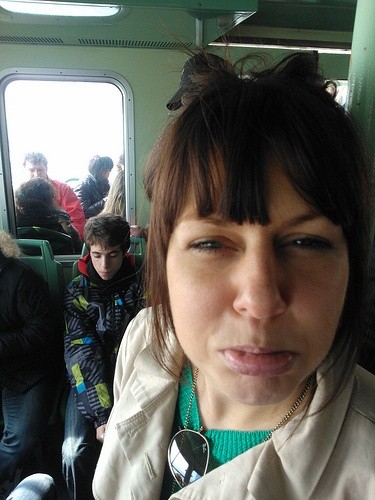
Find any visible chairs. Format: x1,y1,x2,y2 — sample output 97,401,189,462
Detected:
11,238,149,448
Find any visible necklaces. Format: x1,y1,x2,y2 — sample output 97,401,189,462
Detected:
170,367,312,494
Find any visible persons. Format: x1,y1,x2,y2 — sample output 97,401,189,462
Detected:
15,177,83,255
61,213,148,500
166,52,237,111
73,156,126,223
23,153,86,241
91,77,375,500
5,473,56,500
0,230,57,484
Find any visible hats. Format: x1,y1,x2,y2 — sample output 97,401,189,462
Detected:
166,52,232,110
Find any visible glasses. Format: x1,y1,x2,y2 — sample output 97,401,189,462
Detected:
167,425,209,488
